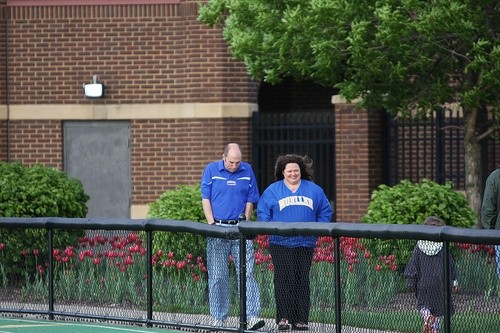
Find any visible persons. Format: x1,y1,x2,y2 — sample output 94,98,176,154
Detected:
404,216,460,333
481,169,500,283
257,155,333,330
200,143,266,331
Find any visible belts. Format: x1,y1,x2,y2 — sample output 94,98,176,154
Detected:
214,218,246,225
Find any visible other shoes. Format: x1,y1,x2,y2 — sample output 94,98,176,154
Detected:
278,318,290,330
423,314,436,333
247,316,265,330
292,322,308,330
211,320,225,327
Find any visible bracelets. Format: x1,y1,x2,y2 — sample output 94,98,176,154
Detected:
209,221,215,225
